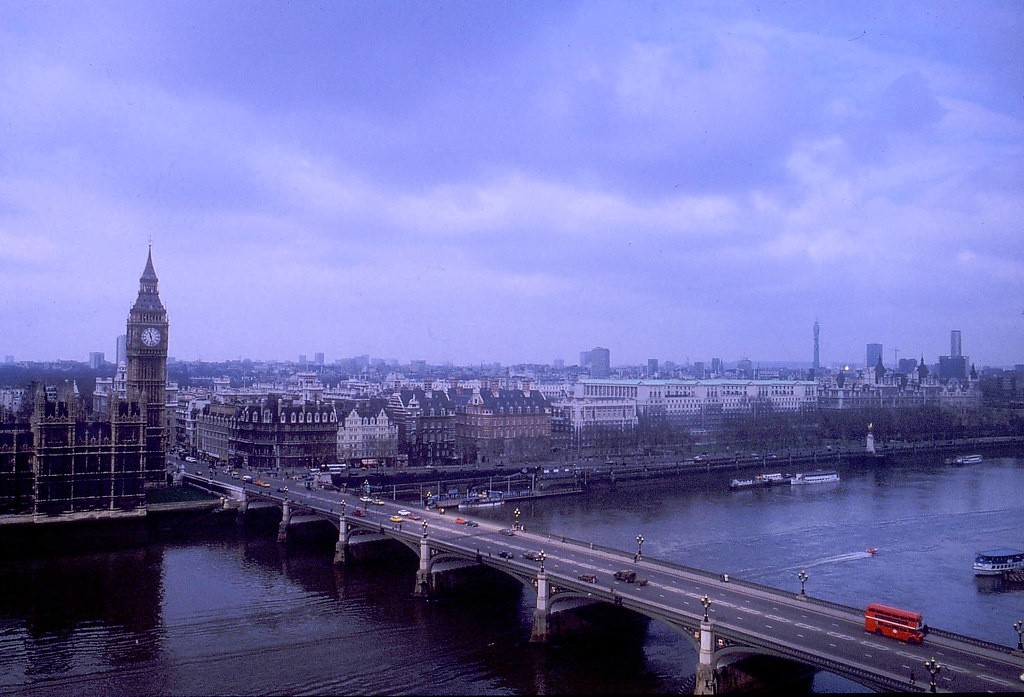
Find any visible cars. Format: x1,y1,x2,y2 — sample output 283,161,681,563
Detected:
398,509,412,516
371,497,384,505
360,496,373,503
455,518,478,527
408,513,421,520
390,515,403,522
523,550,544,561
499,528,514,536
353,507,368,518
498,550,514,559
578,572,599,584
171,446,348,494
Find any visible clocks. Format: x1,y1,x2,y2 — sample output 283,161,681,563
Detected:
140,327,162,347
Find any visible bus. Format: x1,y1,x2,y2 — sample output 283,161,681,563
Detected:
865,603,927,646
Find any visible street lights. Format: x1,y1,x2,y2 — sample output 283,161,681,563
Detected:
1013,620,1024,650
514,506,521,526
700,593,712,622
636,535,644,555
538,549,547,572
925,657,942,694
798,569,808,596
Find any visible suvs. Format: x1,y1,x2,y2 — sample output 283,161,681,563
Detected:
613,569,636,583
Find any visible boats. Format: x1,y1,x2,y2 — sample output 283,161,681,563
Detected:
955,454,984,466
729,470,840,489
972,546,1024,575
